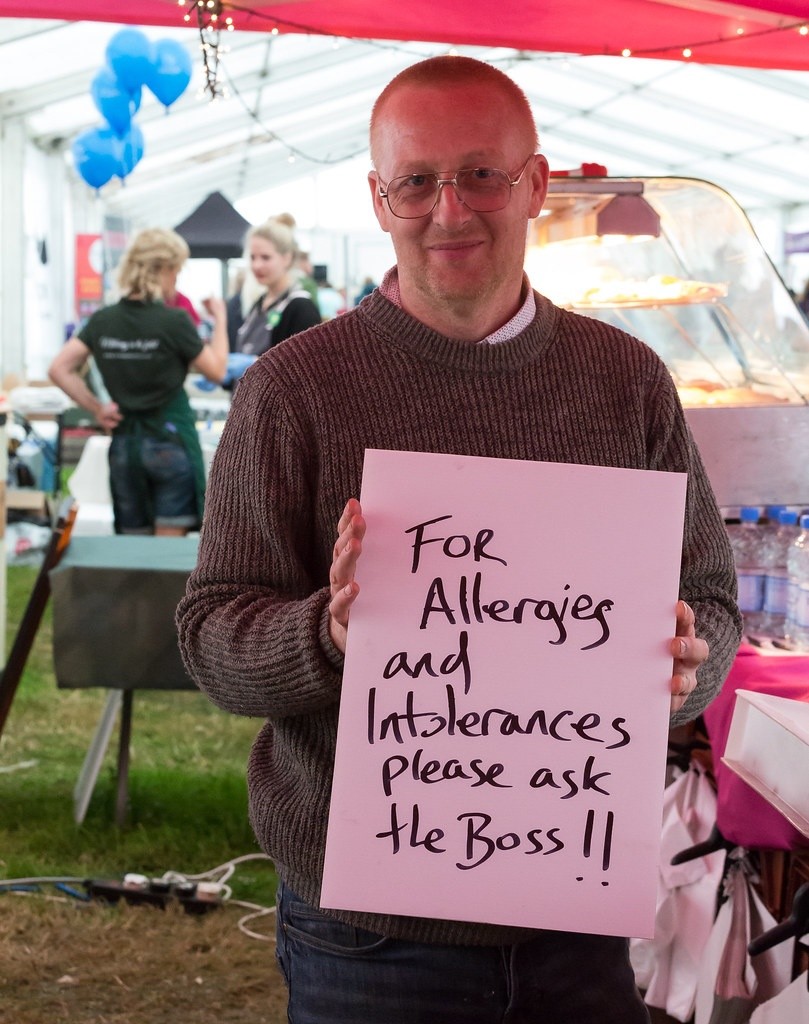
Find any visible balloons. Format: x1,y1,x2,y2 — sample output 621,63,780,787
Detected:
77,25,192,188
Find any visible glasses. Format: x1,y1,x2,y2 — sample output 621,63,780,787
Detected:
376,155,536,221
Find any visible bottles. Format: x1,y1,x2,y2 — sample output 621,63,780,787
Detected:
730,505,809,652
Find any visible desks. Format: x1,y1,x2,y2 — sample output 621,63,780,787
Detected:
54,533,200,824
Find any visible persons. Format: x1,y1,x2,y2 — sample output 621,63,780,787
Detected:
50,225,230,537
167,58,742,1024
239,214,320,356
